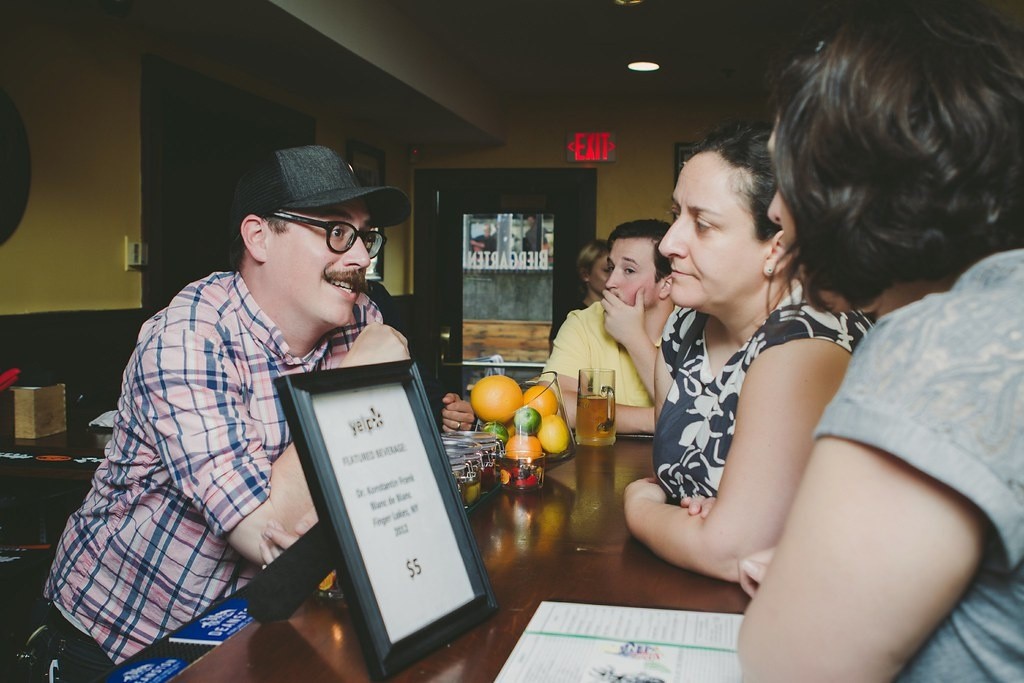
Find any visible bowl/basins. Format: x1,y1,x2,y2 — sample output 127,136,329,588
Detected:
481,371,577,472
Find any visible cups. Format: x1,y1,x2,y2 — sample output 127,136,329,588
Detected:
496,450,546,493
575,367,616,445
441,429,503,508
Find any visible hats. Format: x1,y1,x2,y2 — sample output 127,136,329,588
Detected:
229,144,412,238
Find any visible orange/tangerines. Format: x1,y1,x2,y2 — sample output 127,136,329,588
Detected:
470,376,570,459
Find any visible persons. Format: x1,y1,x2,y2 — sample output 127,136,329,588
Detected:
364,278,475,432
43,142,411,683
621,117,877,583
537,220,672,433
470,214,540,252
735,0,1024,682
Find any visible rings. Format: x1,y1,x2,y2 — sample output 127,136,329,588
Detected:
457,421,461,430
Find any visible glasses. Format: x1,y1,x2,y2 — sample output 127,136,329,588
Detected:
270,211,387,258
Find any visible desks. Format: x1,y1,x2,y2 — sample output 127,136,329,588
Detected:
82,433,762,683
0,410,113,485
